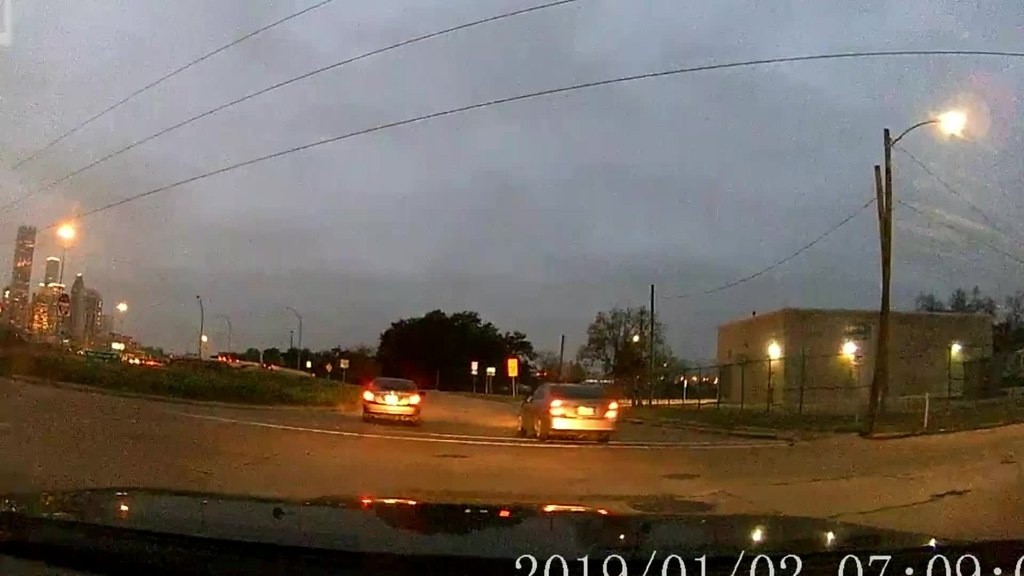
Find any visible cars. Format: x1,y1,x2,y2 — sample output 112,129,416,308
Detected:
518,381,621,442
359,376,427,427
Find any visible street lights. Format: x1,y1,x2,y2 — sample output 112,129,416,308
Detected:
285,306,303,370
857,104,967,437
195,294,204,362
